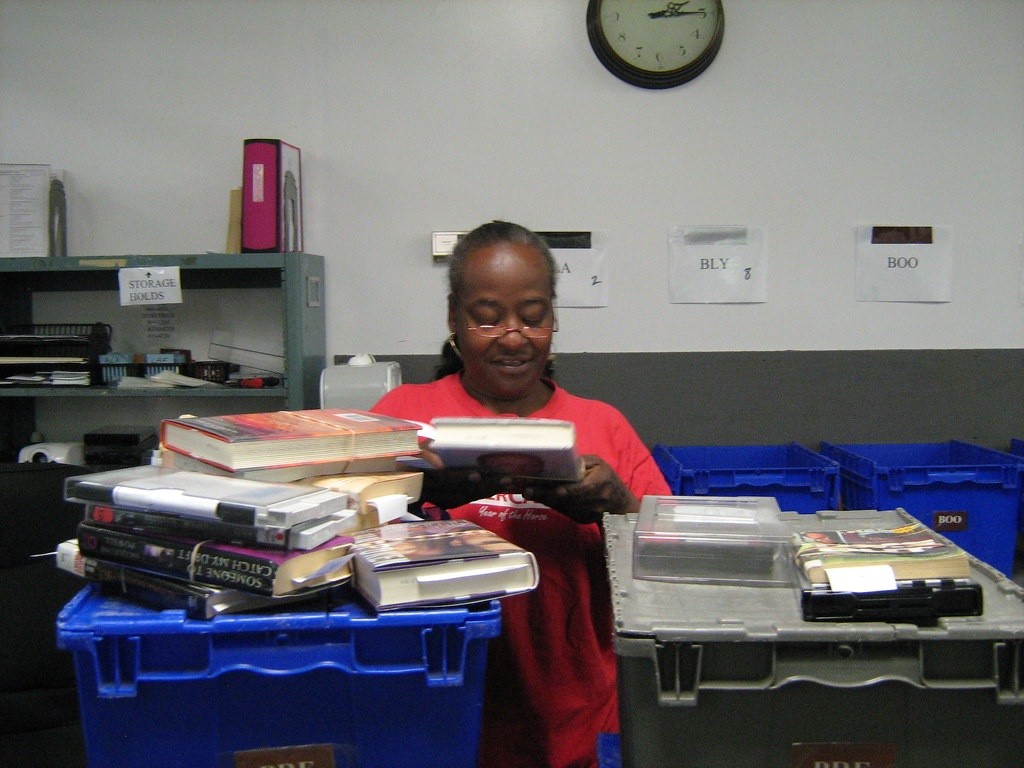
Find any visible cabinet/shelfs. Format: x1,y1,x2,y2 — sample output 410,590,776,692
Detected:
0,252,327,472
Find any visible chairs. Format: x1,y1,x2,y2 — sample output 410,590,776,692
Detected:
0,462,86,735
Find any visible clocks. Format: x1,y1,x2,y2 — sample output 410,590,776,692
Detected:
586,0,725,90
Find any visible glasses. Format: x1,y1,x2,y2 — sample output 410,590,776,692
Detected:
463,314,559,339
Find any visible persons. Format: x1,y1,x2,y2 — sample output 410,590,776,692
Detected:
368,221,676,768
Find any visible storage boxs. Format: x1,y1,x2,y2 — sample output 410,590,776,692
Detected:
609,506,1024,768
650,443,842,515
56,585,502,768
818,440,1024,581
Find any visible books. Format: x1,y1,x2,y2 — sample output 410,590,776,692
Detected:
56,407,424,619
0,322,112,387
341,520,539,612
224,140,302,254
397,417,585,485
793,522,971,584
